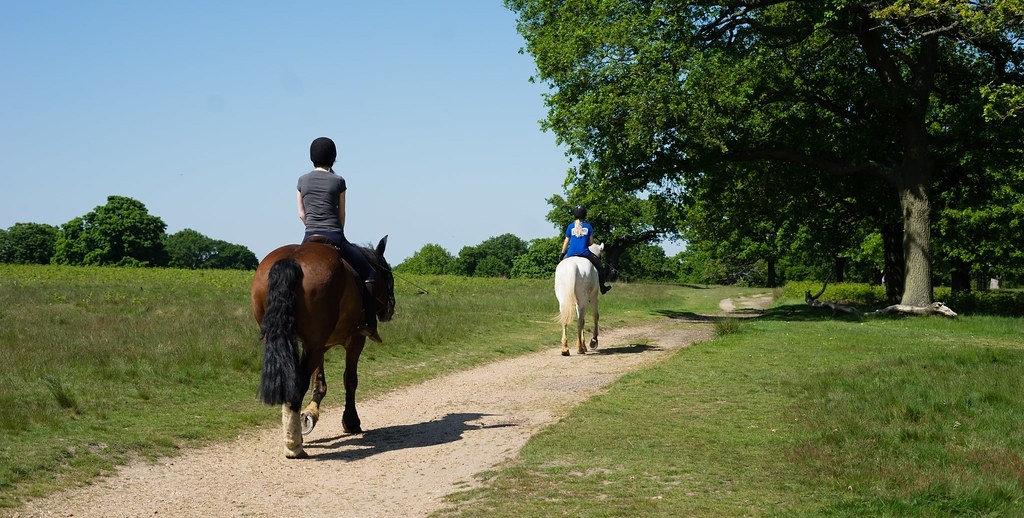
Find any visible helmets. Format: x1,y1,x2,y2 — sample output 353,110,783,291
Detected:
573,205,587,219
310,136,336,161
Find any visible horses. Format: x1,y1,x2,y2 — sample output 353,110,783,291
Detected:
250,234,395,458
554,243,606,356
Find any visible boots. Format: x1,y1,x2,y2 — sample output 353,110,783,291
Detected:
600,283,611,295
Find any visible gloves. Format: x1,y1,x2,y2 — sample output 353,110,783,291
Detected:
559,253,565,261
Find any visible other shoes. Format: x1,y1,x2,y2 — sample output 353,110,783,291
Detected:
358,323,381,343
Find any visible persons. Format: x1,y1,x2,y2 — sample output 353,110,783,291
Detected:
296,137,383,343
559,206,611,294
